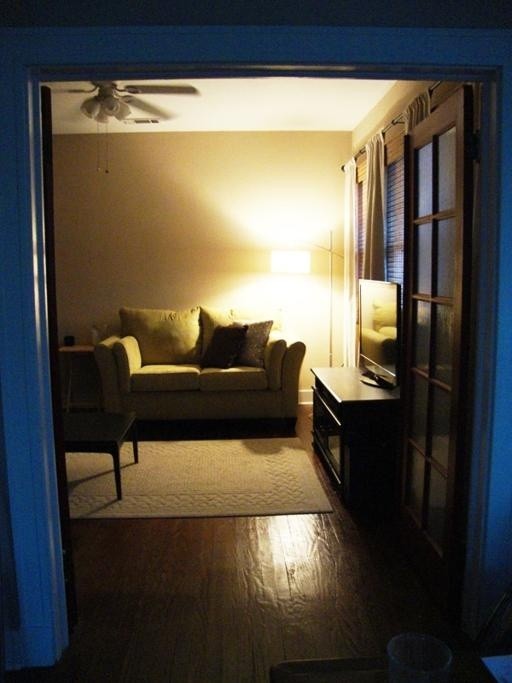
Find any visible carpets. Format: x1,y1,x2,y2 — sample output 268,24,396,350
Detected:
65,437,333,520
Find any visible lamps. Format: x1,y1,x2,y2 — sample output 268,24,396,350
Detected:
80,89,132,124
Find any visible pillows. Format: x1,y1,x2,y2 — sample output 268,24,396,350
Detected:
119,308,201,365
201,326,248,368
200,306,273,367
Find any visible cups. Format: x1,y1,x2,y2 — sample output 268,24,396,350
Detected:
63,336,75,346
386,632,453,683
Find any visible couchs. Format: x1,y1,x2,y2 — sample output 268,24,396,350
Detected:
94,307,306,421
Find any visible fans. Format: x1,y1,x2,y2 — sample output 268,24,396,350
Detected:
51,80,197,121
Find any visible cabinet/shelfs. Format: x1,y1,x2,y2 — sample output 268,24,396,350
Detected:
309,367,401,505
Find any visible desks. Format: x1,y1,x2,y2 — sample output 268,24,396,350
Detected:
58,344,106,414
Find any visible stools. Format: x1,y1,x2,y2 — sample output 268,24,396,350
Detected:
61,411,139,501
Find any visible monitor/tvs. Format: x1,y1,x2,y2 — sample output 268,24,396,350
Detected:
356,280,402,392
